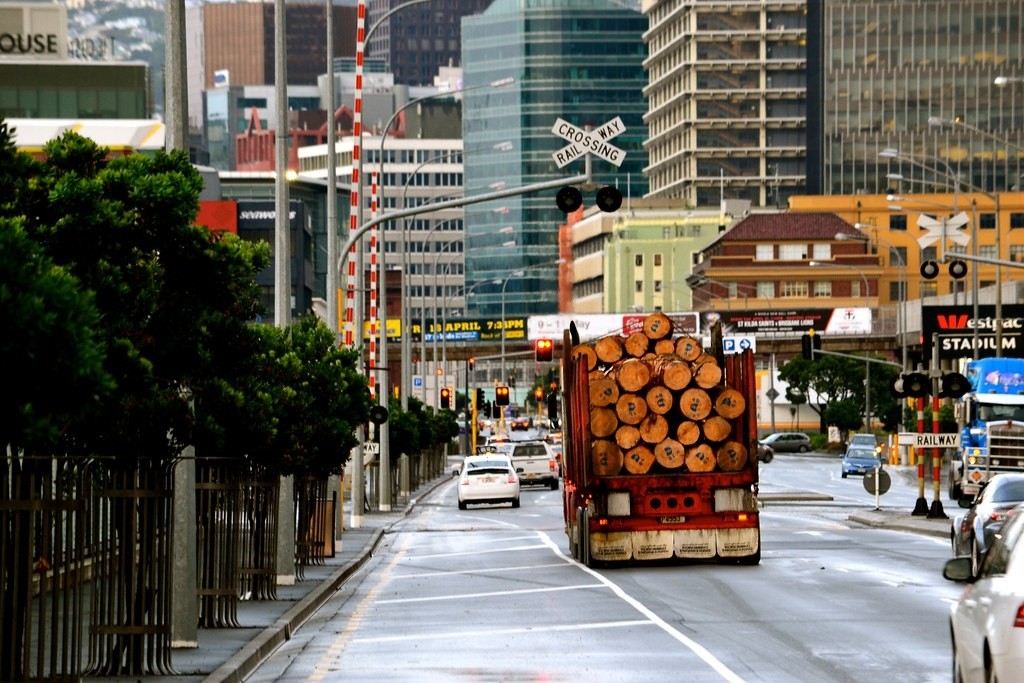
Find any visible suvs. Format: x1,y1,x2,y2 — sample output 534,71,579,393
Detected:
508,440,562,489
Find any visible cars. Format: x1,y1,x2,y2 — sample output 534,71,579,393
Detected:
844,433,885,456
840,446,882,479
455,413,561,471
949,473,1024,578
452,454,524,509
759,432,813,454
943,504,1024,683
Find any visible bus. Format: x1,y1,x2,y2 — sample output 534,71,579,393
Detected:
945,394,1024,510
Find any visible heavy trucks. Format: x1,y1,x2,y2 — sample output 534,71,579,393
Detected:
563,320,764,567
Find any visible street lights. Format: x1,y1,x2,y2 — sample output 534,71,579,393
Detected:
502,259,567,392
376,76,518,515
885,172,981,364
405,180,527,494
834,232,908,466
808,260,871,436
857,222,926,345
878,151,1001,358
400,141,515,499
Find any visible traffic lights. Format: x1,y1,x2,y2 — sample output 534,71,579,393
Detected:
511,377,517,389
549,381,558,395
508,376,513,387
370,406,390,428
596,187,622,214
536,387,544,402
483,400,492,419
903,373,934,396
492,400,501,421
556,186,582,212
440,388,449,409
496,386,509,408
537,339,555,364
942,371,972,400
469,357,474,371
476,387,485,411
879,405,912,434
